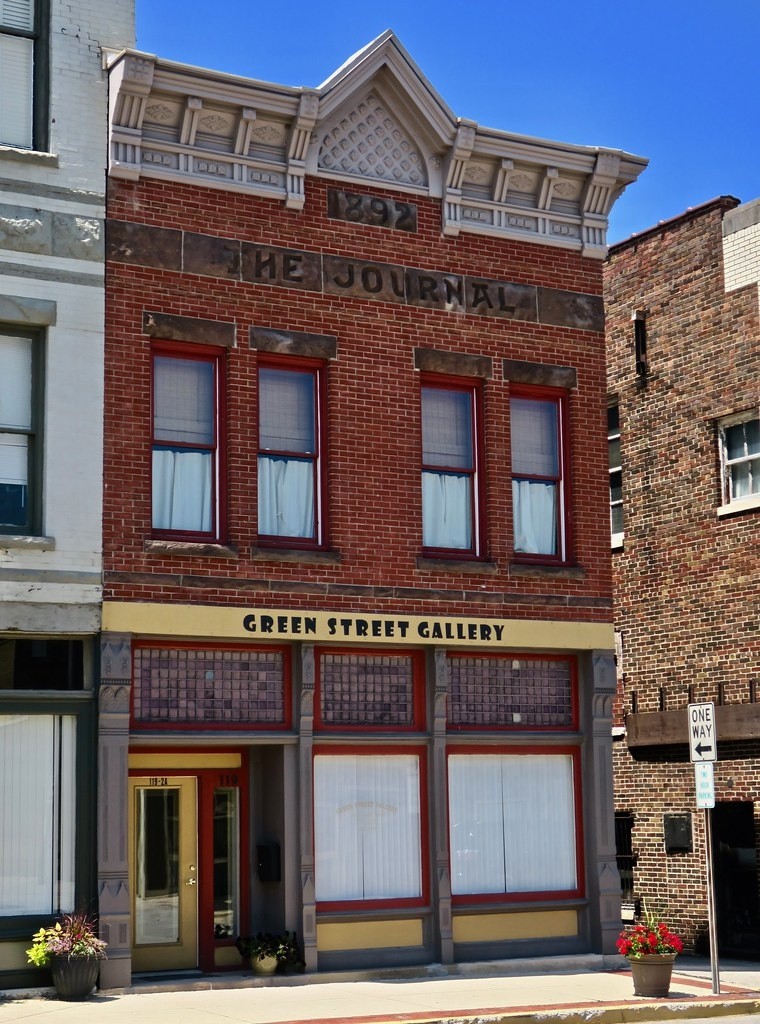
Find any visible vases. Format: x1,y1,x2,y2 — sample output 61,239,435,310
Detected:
625,953,678,996
50,954,102,1002
251,950,278,975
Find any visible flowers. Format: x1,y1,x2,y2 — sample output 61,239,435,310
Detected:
616,895,682,957
235,931,296,960
25,898,107,967
